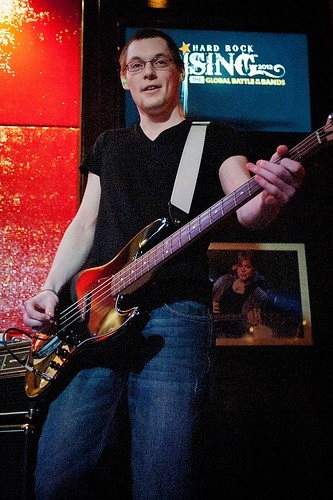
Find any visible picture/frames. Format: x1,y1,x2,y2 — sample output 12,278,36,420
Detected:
207,243,313,346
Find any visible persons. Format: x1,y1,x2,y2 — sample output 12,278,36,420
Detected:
211,250,270,339
21,26,307,500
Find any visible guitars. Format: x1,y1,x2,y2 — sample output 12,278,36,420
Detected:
23,111,333,400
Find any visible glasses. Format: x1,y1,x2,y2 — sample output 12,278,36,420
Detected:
126,56,175,72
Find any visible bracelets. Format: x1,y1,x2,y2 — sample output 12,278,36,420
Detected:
40,289,57,295
